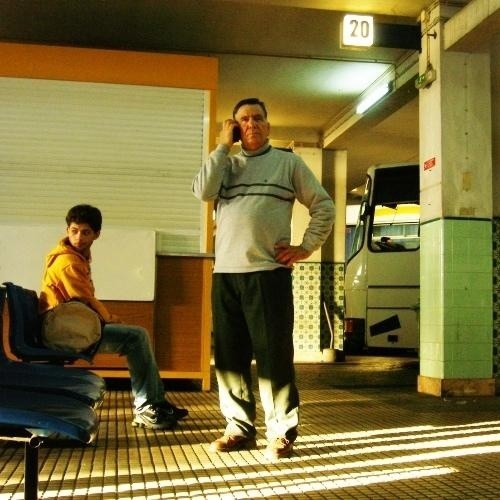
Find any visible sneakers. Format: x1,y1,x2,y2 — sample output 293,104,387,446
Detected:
155,399,189,420
267,436,295,458
132,406,178,432
208,433,257,453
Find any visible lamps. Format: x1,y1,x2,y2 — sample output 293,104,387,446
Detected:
355,81,392,115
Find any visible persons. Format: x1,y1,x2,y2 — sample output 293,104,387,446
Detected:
37,203,190,431
190,96,336,461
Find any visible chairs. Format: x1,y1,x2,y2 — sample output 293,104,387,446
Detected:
0,282,107,500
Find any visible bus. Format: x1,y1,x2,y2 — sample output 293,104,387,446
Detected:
343,161,420,358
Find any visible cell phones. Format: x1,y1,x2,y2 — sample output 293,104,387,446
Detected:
232,127,240,142
